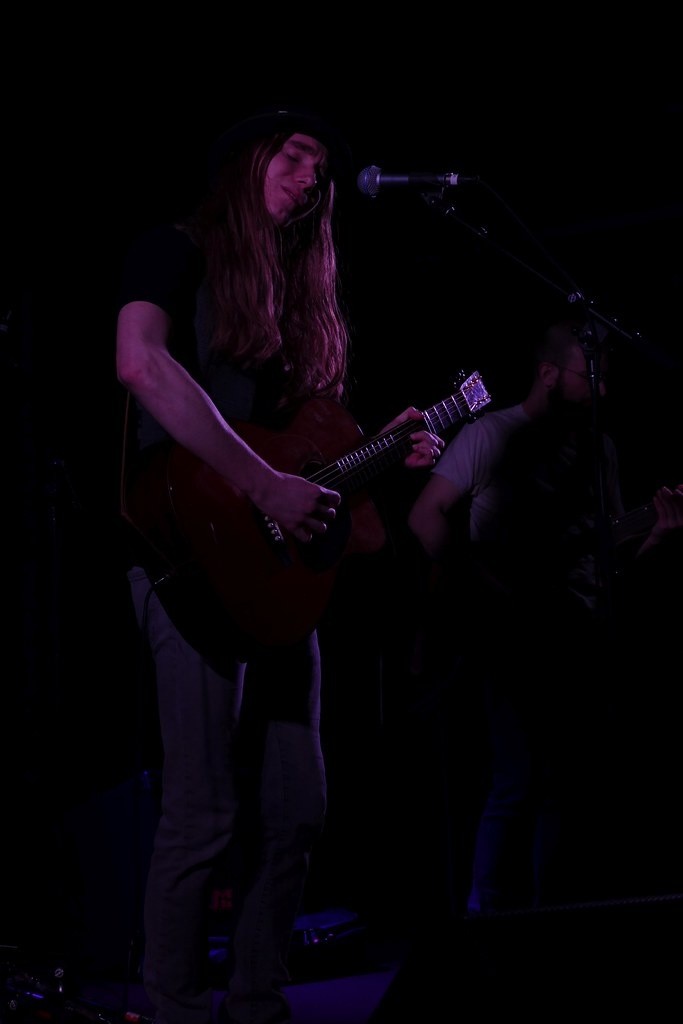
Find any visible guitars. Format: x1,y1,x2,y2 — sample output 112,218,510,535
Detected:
157,369,488,640
431,499,661,641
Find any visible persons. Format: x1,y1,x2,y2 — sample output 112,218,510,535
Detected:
404,300,683,1023
109,101,450,1023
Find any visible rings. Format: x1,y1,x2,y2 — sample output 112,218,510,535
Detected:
303,528,313,546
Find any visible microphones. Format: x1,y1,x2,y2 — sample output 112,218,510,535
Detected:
358,165,482,197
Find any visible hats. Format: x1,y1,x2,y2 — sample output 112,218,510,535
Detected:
210,102,354,182
